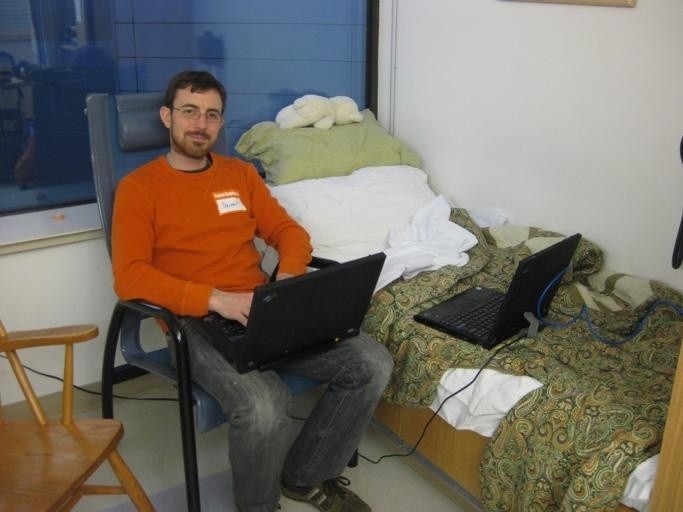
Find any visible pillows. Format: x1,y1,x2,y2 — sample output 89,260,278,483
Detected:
233,107,424,186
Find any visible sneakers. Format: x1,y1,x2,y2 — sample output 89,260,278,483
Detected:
279,474,371,511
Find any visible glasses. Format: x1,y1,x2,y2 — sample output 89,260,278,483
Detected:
171,106,223,124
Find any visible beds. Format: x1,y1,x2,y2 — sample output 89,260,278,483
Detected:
256,170,683,510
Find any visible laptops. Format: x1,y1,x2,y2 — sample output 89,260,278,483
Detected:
413,233,582,351
190,251,387,375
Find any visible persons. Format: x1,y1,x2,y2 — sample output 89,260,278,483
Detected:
110,70,394,512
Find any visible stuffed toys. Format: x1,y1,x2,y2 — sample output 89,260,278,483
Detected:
274,95,363,129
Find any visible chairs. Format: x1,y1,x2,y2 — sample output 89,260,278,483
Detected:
0,316,154,511
83,90,359,512
